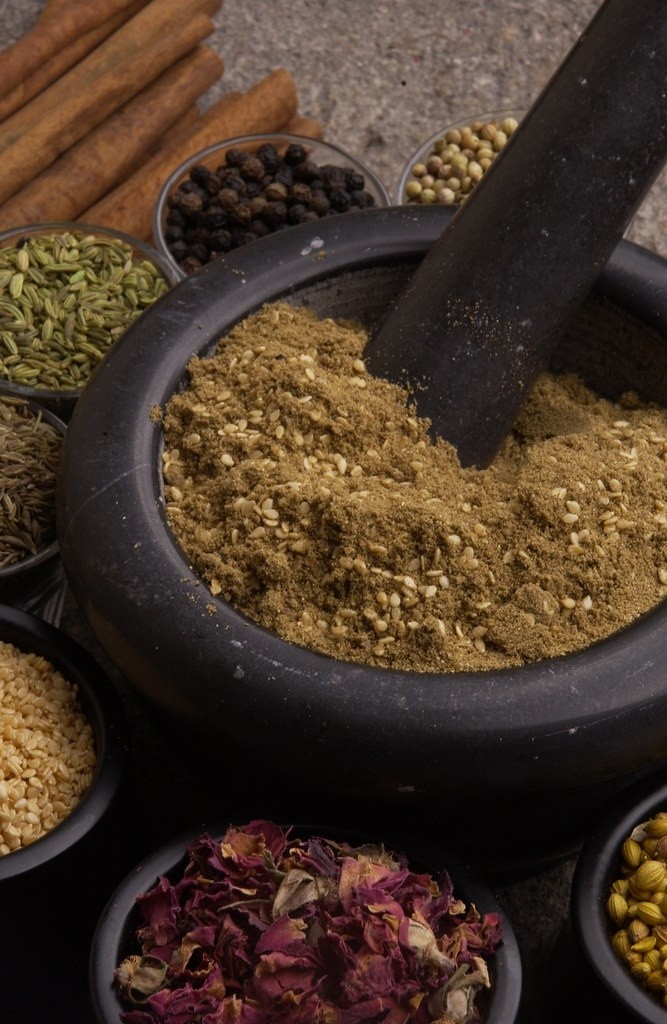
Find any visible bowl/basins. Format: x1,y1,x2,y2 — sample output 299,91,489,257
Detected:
394,107,539,206
88,813,523,1024
570,783,667,1024
150,133,389,282
0,394,69,590
58,207,667,819
0,223,180,410
0,602,129,887
